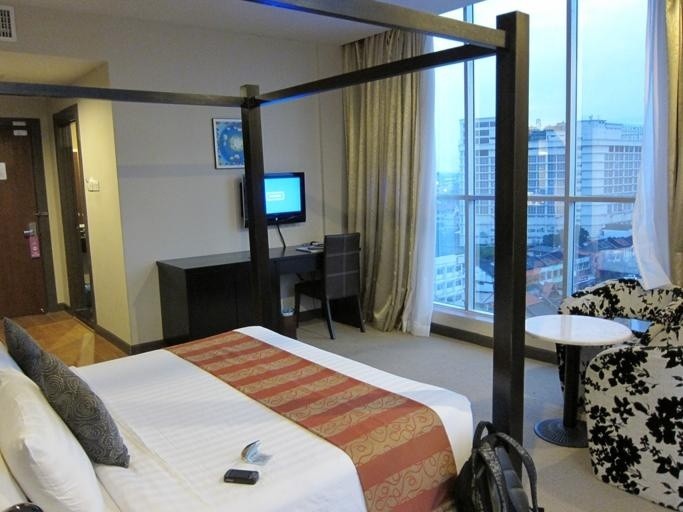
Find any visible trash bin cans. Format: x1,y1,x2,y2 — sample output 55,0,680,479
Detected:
280,309,297,340
84,273,92,307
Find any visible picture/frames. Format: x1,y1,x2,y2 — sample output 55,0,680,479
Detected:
212,118,245,170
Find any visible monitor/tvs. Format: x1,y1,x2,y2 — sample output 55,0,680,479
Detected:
242,172,306,227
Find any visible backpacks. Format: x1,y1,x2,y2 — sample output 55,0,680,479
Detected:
451,420,538,508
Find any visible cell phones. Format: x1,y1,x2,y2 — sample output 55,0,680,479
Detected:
224,469,259,484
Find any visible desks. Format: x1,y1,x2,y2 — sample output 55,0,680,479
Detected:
154,244,324,345
526,314,632,451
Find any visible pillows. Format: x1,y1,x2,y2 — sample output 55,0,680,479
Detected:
0,315,130,512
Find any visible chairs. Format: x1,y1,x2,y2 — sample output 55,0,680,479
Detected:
293,231,366,339
555,276,682,511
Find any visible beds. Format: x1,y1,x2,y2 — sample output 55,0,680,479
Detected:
65,323,474,512
